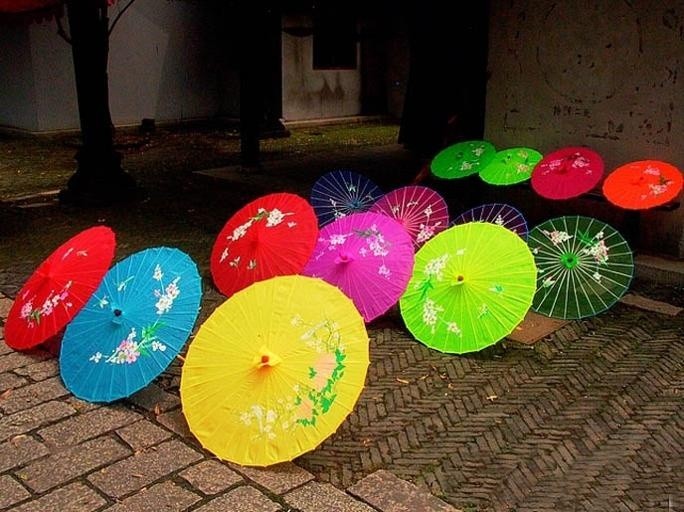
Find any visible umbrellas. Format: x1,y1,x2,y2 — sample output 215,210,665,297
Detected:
179,275,370,467
59,246,202,403
4,226,116,350
